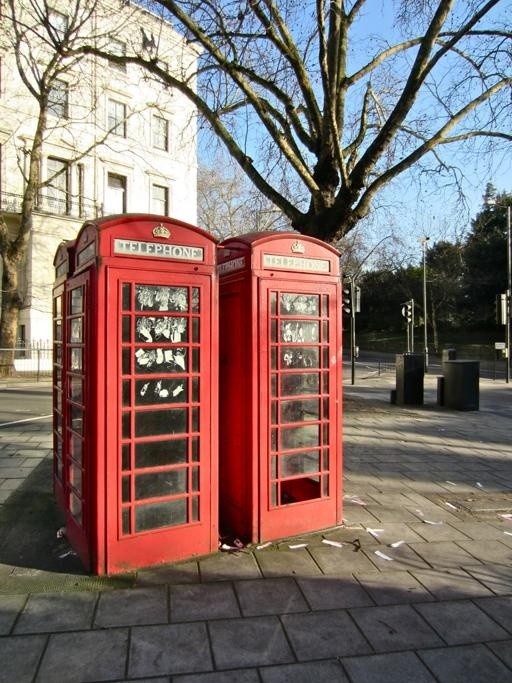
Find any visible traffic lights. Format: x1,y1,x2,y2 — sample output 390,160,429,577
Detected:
492,294,506,325
404,302,414,324
342,281,351,319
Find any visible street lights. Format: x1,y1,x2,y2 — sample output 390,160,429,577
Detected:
418,235,430,371
485,197,512,382
